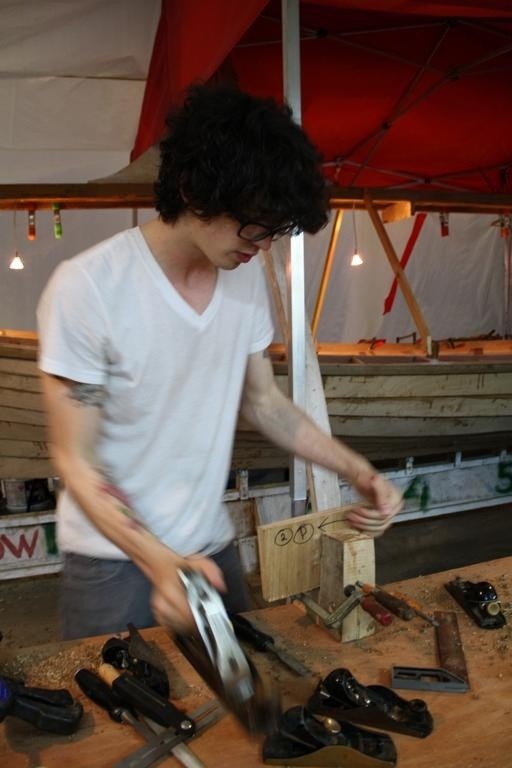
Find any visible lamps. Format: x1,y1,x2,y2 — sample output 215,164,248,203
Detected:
9,205,24,270
350,203,363,266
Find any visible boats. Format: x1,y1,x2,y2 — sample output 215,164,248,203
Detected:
0,183,512,482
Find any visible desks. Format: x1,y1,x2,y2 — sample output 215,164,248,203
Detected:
0,555,512,768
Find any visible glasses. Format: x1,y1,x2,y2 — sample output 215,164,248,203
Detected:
225,208,296,246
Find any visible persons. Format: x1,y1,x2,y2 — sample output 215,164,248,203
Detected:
36,78,404,640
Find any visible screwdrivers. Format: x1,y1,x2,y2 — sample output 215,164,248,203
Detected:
72,665,172,757
